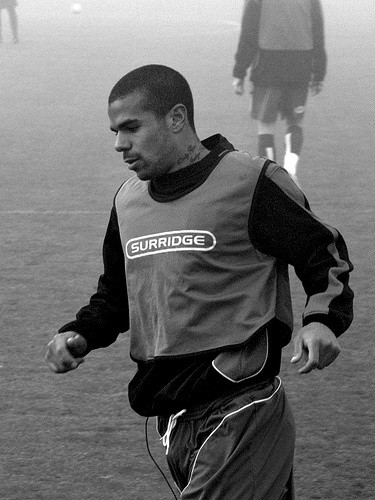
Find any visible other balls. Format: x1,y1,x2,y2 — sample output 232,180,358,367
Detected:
72,4,82,13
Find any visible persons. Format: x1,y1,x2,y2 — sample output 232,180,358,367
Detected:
232,0,328,189
0,0,19,43
44,63,356,500
